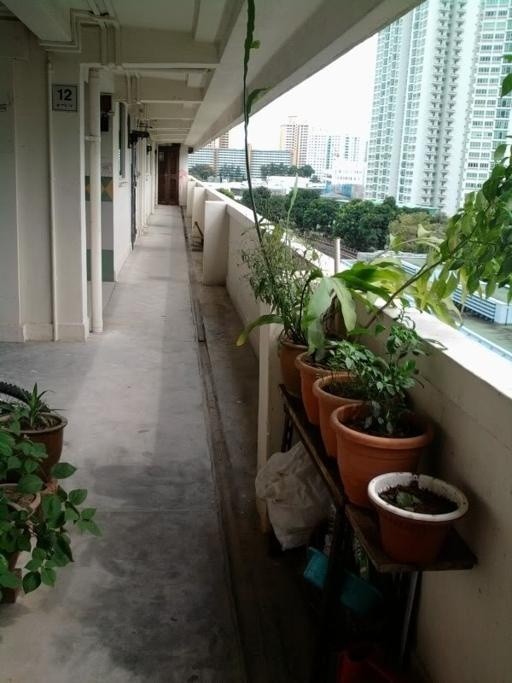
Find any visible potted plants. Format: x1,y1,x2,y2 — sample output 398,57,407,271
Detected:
368,470,468,560
1,383,102,606
332,309,437,509
232,0,509,425
313,323,402,457
